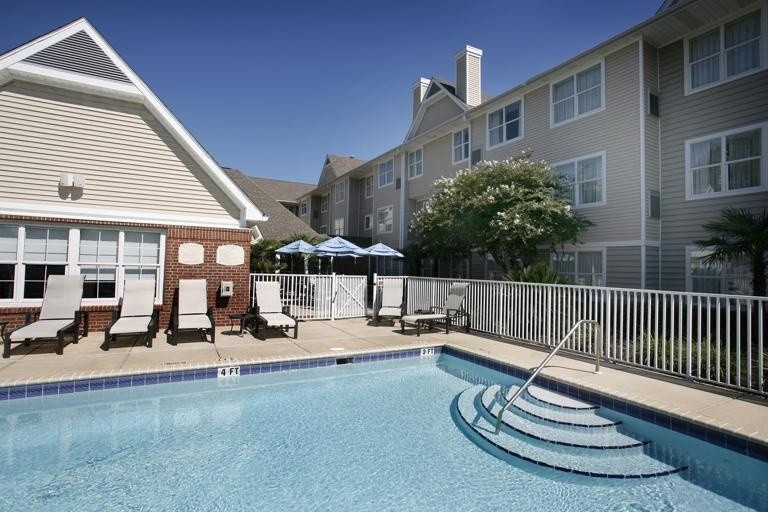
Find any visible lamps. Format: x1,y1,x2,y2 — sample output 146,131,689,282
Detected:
60,175,74,188
74,175,85,188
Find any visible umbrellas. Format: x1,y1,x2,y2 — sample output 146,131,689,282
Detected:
275,240,318,276
356,242,404,276
312,236,369,276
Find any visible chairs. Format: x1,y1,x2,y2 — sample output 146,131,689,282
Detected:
399,282,469,336
250,281,299,341
171,279,215,345
4,274,88,358
105,279,159,351
374,278,407,328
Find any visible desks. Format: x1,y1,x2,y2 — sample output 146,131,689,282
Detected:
414,310,431,315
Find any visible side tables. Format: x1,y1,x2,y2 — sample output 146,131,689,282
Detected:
0,320,9,342
229,314,256,336
